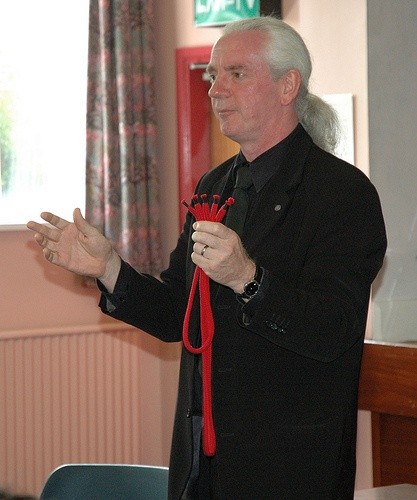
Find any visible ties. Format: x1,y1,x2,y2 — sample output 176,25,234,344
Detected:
225,166,253,237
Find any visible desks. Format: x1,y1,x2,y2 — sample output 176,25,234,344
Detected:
358,340,417,488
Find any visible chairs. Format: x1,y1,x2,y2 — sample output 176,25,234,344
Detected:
40,464,169,500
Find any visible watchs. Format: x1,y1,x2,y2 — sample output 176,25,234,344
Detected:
235,263,263,300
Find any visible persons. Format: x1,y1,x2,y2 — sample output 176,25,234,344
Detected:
26,17,388,500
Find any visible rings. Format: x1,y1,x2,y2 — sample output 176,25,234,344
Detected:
201,245,209,255
